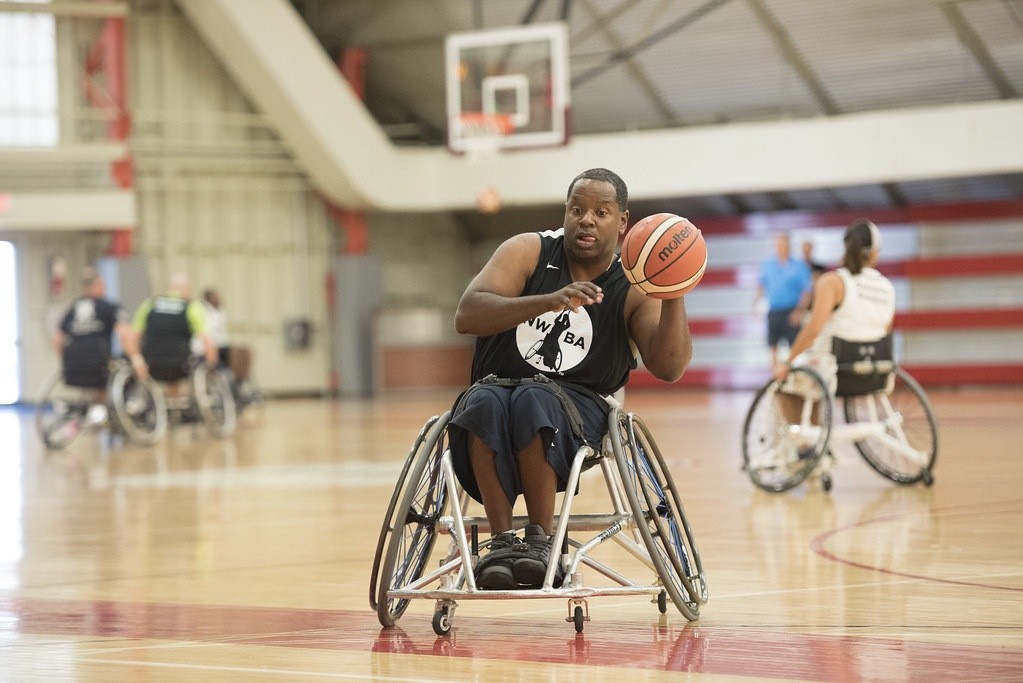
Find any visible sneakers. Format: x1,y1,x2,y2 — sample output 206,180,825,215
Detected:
475,533,522,588
510,523,564,585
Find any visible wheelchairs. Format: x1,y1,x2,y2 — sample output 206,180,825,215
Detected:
366,376,708,637
732,355,940,494
36,349,261,448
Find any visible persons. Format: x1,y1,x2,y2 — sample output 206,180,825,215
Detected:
453,167,701,590
50,266,256,407
753,218,896,458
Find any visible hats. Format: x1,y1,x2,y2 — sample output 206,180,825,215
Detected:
844,220,880,252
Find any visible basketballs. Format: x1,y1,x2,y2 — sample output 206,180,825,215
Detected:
621,212,710,301
